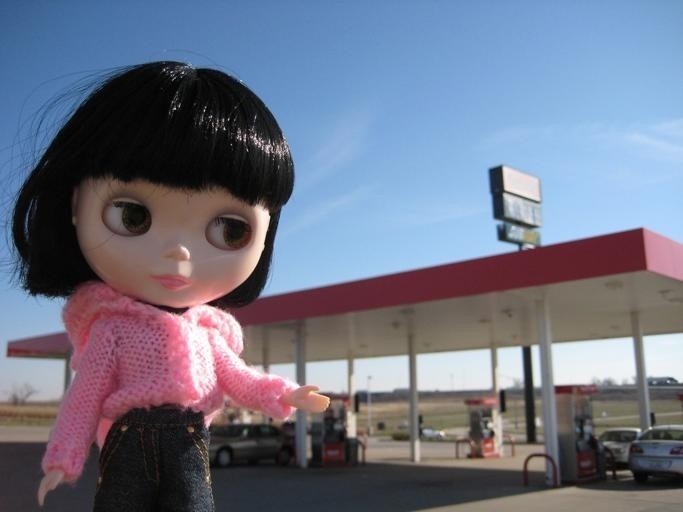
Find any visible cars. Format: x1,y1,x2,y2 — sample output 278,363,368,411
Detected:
598,424,683,487
210,424,295,469
393,423,446,441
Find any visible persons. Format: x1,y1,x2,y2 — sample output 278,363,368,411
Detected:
10,57,332,511
